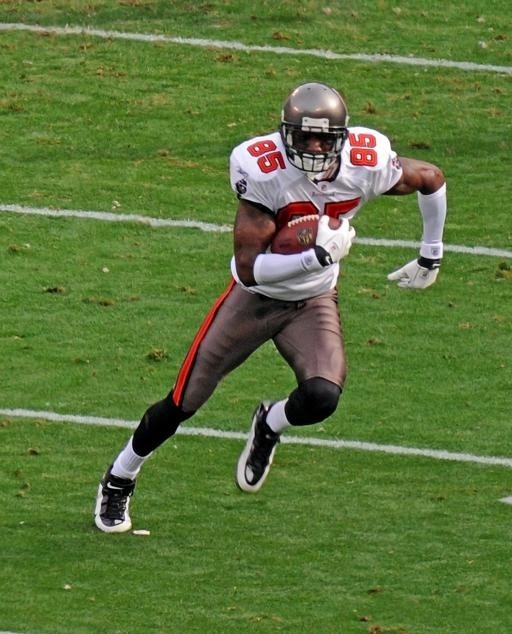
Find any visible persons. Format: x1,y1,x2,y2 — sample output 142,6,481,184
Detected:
94,82,447,532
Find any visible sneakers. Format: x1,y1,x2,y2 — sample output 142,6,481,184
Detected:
92,461,136,536
232,398,282,494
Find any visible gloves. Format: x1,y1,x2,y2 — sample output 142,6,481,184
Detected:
385,255,441,291
314,215,356,265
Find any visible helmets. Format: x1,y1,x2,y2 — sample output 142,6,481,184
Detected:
275,79,352,174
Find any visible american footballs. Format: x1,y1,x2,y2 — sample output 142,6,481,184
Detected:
271,215,341,255
